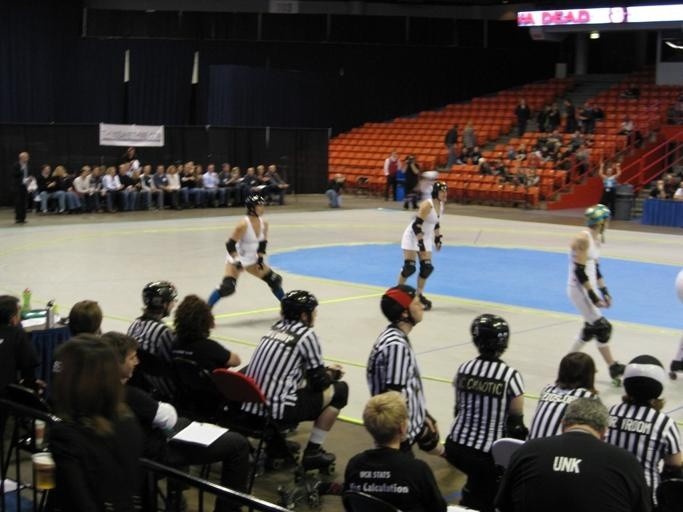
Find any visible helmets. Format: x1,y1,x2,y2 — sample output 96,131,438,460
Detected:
434,182,447,191
471,315,509,349
143,281,178,304
247,195,267,206
281,290,318,315
585,204,610,225
381,284,416,321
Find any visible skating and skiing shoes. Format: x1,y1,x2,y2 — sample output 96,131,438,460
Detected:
610,365,625,385
418,293,431,310
295,446,336,482
669,360,683,379
267,440,300,458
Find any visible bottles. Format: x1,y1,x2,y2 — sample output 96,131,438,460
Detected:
22,288,31,311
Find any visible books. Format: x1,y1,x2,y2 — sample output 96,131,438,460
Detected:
165,420,229,447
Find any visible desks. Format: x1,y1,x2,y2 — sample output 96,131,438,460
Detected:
15,316,85,388
641,198,683,226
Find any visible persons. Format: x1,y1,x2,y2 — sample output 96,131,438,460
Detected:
650,178,667,200
43,338,145,512
670,272,682,383
1,291,39,443
66,303,103,353
37,159,290,214
402,153,420,210
366,286,444,458
604,354,682,511
325,173,346,208
129,280,177,368
561,201,627,384
237,290,350,481
343,389,450,511
443,125,459,171
445,314,529,506
503,399,654,512
527,352,602,450
598,160,621,219
383,150,401,202
123,148,138,162
479,90,638,190
207,196,284,326
673,179,683,201
102,331,144,426
665,174,678,199
11,152,34,226
399,182,448,309
171,293,241,389
461,120,477,154
